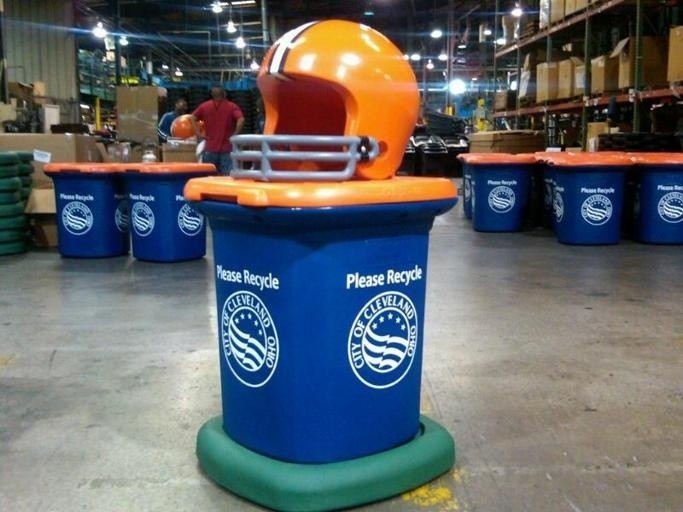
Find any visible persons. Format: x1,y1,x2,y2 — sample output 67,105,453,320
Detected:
255,96,265,133
155,97,187,143
189,83,242,179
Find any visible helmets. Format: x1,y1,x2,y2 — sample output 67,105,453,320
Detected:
170,113,206,141
254,20,421,179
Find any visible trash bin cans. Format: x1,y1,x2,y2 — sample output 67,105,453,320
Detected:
42,163,217,263
182,176,459,465
456,152,683,246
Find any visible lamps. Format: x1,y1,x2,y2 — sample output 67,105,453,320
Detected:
207,1,263,73
89,1,184,79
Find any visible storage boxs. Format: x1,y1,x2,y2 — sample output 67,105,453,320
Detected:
465,0,683,155
0,77,199,253
